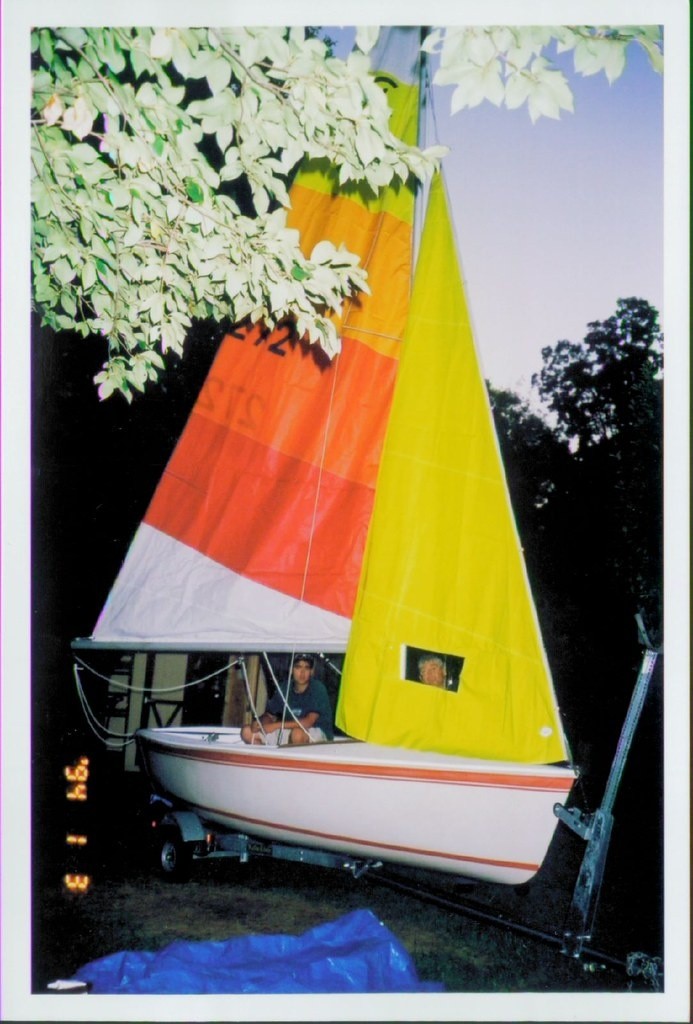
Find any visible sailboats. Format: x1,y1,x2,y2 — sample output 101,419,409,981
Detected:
67,26,586,900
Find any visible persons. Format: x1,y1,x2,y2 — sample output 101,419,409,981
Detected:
418,656,446,686
240,653,335,748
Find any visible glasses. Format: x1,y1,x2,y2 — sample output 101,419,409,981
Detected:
295,654,314,660
